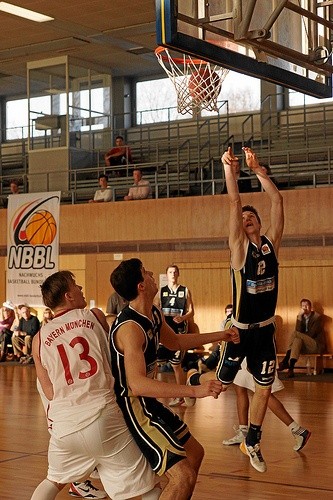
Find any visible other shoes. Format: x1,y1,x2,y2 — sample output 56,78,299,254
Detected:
284,370,295,379
276,362,289,371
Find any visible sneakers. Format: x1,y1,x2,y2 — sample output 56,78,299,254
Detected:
239,437,267,473
89,468,99,478
293,429,311,451
183,368,199,407
168,398,184,406
68,482,107,500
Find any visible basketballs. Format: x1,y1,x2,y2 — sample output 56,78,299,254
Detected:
188,68,221,103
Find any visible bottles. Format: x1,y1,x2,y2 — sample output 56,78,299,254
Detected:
306,357,312,376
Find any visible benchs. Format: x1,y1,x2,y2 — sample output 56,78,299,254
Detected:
274,352,333,375
1,105,332,193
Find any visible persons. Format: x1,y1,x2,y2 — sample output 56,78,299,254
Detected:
0,290,234,479
30,271,163,500
277,298,327,379
0,181,21,208
222,356,312,451
106,136,134,178
124,169,153,200
154,264,194,406
184,146,285,473
68,258,241,500
89,174,113,203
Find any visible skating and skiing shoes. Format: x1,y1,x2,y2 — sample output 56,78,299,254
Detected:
222,433,247,445
